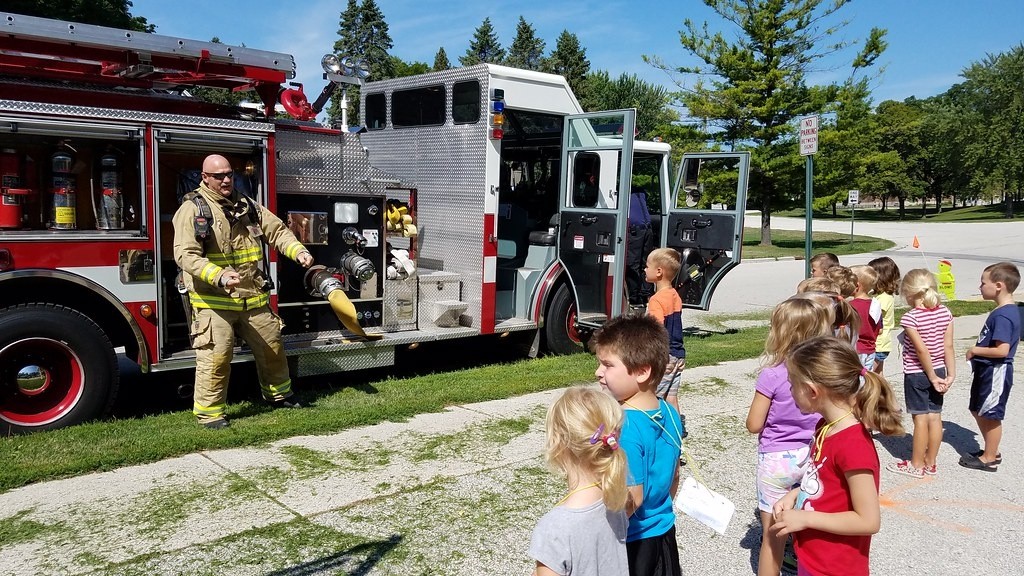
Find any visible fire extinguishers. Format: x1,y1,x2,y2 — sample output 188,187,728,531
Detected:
0,141,26,228
40,137,79,230
89,142,137,230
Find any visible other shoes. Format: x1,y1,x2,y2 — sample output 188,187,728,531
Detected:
758,532,792,550
781,554,797,574
274,396,310,408
922,455,937,475
204,419,230,429
887,459,925,479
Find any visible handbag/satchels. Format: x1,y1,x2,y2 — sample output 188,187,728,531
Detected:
674,476,735,536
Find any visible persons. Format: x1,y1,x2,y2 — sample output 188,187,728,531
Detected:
590,168,653,308
959,262,1021,471
769,335,905,576
848,267,883,437
868,256,900,377
173,154,314,428
790,253,860,350
594,315,683,576
645,249,687,465
886,269,956,478
531,388,630,576
745,298,832,576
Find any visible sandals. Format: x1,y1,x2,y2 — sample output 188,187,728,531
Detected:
958,456,997,472
965,449,1001,465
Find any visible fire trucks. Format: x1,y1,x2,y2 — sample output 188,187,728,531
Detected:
0,11,752,445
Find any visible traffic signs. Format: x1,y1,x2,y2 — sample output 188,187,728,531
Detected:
848,190,859,205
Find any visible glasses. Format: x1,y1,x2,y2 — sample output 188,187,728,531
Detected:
204,170,234,180
